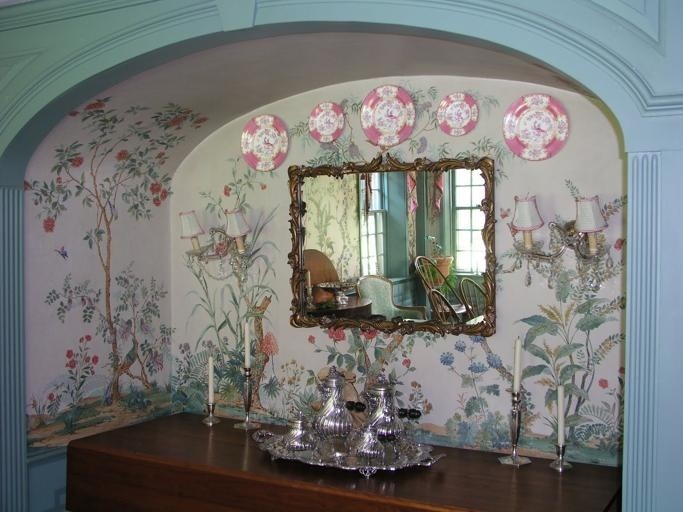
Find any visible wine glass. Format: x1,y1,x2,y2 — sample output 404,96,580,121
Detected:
316,280,356,306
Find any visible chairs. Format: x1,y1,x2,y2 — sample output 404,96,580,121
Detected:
357,255,486,324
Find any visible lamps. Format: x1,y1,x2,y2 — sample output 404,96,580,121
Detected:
512,196,608,260
179,211,251,261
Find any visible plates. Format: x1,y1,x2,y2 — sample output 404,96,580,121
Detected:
240,114,289,173
308,102,346,144
502,92,570,162
360,85,416,148
437,92,479,136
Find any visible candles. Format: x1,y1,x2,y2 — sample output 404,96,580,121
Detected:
557,382,566,445
245,322,250,367
208,354,214,403
513,335,522,394
307,268,310,286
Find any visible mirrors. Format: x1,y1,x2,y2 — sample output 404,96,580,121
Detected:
287,153,496,337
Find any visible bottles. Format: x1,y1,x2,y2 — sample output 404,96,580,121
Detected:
365,375,404,443
314,367,353,442
357,425,385,459
285,411,315,453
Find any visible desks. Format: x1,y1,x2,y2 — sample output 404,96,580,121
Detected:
67,413,622,512
309,297,372,318
